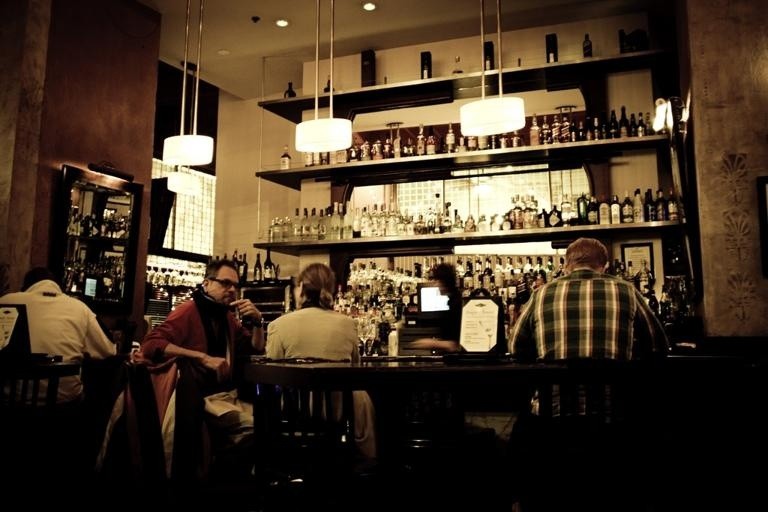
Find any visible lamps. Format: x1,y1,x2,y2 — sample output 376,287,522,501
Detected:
160,0,216,168
293,0,353,154
165,59,201,197
457,0,526,139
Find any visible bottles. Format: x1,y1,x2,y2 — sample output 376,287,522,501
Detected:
145,264,205,288
325,80,335,93
214,250,278,283
268,105,683,298
388,326,399,357
582,34,593,59
284,80,295,98
452,56,464,75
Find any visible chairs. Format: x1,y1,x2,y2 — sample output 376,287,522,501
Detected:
0,344,641,510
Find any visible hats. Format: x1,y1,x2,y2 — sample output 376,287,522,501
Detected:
21,266,62,292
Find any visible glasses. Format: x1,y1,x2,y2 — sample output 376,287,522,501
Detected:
208,278,240,290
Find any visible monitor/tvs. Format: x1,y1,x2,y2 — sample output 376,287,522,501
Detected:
416,283,450,315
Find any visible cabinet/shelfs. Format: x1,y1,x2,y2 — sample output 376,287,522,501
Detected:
238,285,290,344
250,45,688,257
50,163,145,314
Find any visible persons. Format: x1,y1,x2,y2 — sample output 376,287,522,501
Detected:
407,262,464,351
143,257,265,462
0,267,133,459
507,237,669,417
266,262,376,460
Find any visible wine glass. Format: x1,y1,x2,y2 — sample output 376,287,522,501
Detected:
351,315,376,357
